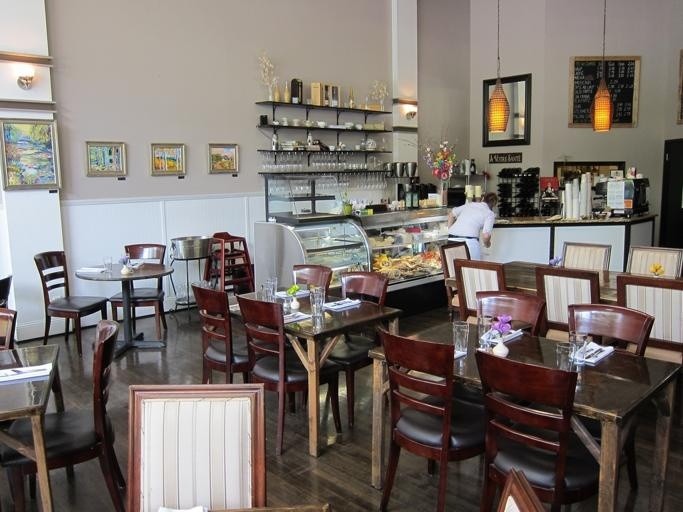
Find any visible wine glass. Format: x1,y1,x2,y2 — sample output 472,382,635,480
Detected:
269,172,388,195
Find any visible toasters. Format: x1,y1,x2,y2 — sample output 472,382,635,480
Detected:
275,81,280,102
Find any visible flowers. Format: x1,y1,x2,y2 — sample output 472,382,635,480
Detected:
398,128,459,182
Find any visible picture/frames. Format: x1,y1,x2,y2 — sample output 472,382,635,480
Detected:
0,118,63,192
482,73,531,147
150,143,186,176
553,161,625,180
85,141,127,177
208,143,239,174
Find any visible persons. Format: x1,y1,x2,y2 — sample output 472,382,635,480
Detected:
446,190,498,277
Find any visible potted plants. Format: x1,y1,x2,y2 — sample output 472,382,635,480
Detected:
340,193,352,215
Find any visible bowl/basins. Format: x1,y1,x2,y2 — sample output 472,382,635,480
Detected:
317,122,326,127
329,146,335,151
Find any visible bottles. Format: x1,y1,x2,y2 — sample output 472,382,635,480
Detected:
349,86,355,109
257,151,363,172
404,184,420,208
284,80,290,102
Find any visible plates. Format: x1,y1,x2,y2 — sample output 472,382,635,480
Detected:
328,124,346,129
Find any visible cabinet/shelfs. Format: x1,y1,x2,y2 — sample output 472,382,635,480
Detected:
255,100,393,225
253,206,452,319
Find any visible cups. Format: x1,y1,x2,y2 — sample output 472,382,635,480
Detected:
478,316,494,349
556,342,574,375
562,172,593,218
257,277,325,317
355,145,360,150
453,321,469,354
345,203,352,215
569,332,587,366
104,253,130,273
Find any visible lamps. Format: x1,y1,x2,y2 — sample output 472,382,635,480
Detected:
17,68,34,90
403,105,418,120
487,0,510,132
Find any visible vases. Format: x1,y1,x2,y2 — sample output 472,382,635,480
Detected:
589,0,614,133
437,182,449,206
